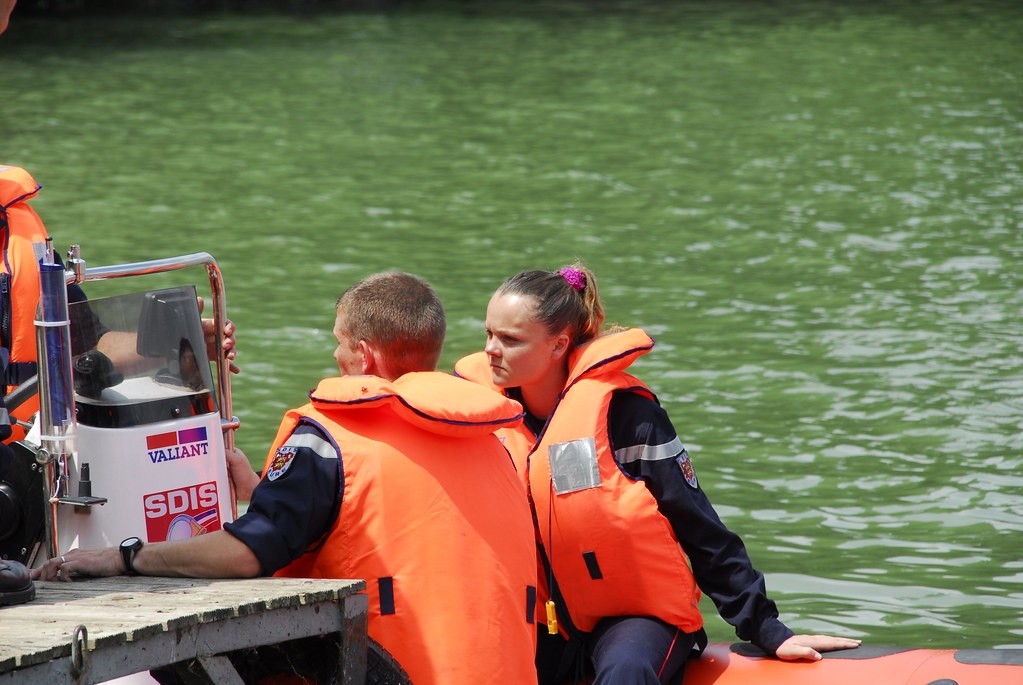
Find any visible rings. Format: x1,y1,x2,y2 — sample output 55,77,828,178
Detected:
60,555,66,562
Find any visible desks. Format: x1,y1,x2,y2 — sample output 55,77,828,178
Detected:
0,576,370,685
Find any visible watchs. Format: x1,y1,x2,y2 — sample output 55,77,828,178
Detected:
119,537,144,577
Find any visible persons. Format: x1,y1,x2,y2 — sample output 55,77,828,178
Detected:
38,272,540,685
225,261,863,685
0,164,239,607
177,337,218,415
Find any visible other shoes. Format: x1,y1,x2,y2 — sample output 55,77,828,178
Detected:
0,557,35,607
